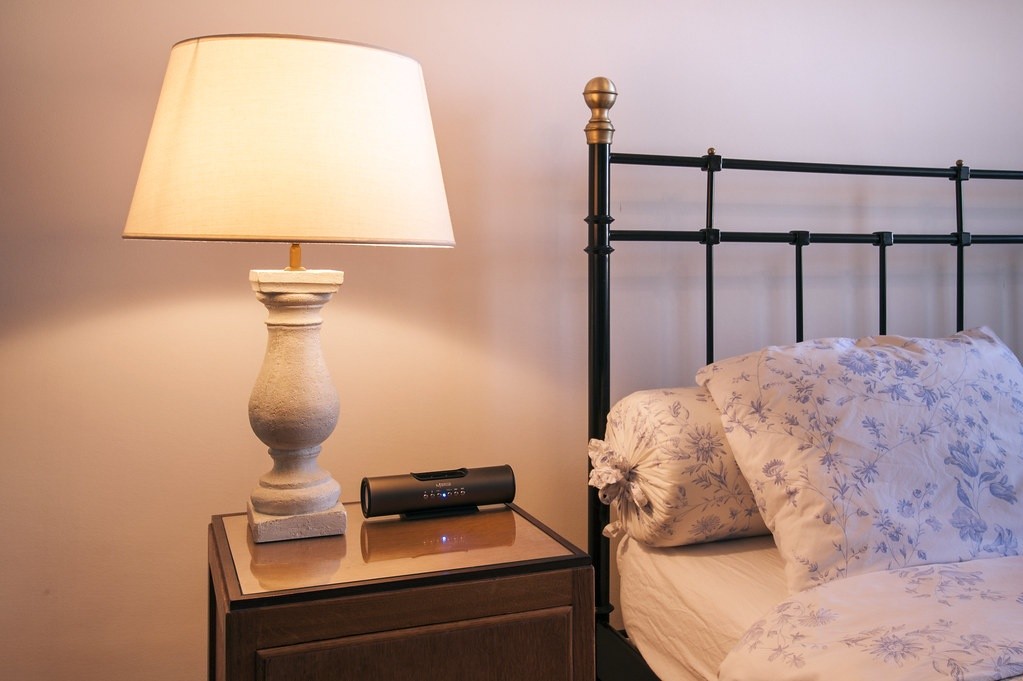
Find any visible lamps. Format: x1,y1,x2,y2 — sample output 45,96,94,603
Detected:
121,33,453,544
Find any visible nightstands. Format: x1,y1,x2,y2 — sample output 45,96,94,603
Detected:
208,495,601,681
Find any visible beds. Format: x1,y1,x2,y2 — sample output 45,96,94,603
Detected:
582,76,1023,681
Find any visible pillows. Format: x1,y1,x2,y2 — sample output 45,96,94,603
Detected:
588,389,775,548
696,327,1023,592
